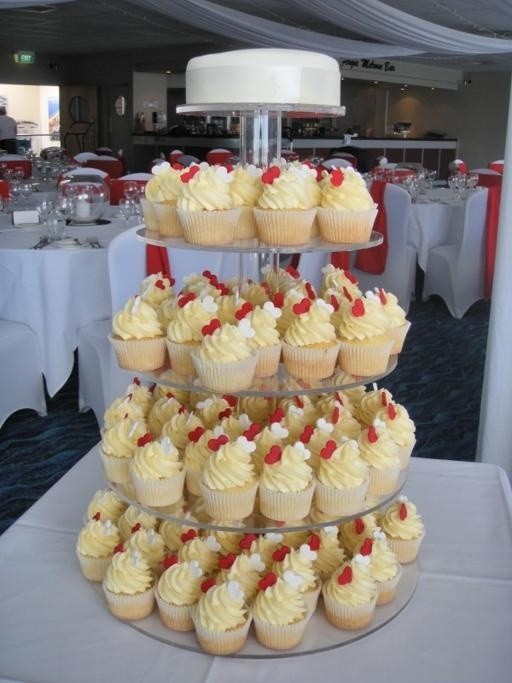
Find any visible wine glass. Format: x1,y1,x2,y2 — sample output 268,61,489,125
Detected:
118,180,146,226
362,168,438,202
447,172,479,201
38,200,64,242
2,166,33,204
32,155,69,188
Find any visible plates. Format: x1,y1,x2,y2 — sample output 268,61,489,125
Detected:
48,239,84,250
14,222,45,229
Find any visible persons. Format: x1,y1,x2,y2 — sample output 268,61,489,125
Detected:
0,106,17,154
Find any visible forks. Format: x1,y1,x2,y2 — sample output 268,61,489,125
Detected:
93,237,104,249
85,236,97,249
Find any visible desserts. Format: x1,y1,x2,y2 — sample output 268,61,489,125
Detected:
74,489,423,655
99,364,419,521
106,264,411,393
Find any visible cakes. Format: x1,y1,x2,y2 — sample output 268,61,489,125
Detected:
142,160,378,246
184,46,343,105
